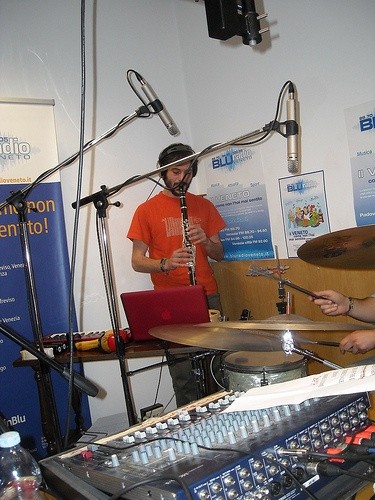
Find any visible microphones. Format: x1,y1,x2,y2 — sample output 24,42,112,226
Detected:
136,73,177,136
286,82,299,173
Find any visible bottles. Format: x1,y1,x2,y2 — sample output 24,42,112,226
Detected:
0,431,54,500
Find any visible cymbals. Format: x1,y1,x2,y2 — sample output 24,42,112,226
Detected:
199,310,368,334
147,322,297,355
294,222,375,271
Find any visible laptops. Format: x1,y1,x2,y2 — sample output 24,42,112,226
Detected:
121,286,210,344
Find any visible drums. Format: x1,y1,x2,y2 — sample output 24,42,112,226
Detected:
217,348,310,389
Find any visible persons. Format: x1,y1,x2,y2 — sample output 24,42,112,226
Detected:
307,289,375,356
126,142,228,409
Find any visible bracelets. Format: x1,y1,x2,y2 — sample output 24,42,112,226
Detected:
160,258,167,273
343,297,354,315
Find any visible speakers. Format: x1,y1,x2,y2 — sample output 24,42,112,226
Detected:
205,0,240,40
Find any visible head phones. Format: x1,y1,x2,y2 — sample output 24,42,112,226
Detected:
156,146,197,178
